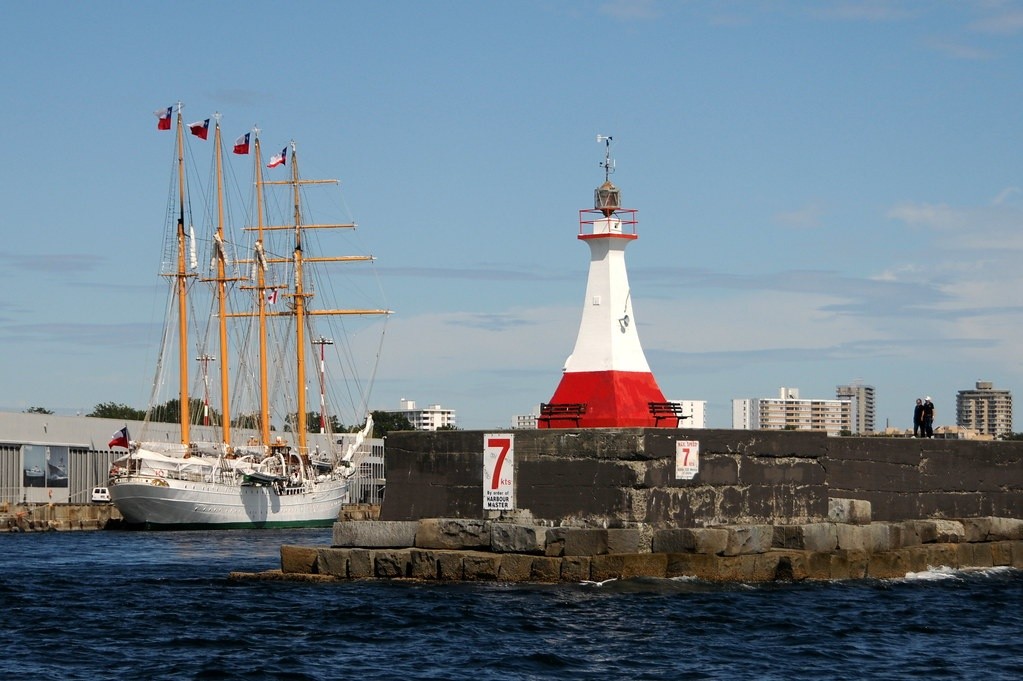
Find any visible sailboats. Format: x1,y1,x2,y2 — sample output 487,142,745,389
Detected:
110,100,396,530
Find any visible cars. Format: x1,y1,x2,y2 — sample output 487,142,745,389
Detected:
91,487,112,503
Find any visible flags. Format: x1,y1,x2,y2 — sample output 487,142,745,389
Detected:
268,291,277,305
186,118,210,140
267,146,287,168
158,106,172,130
233,132,250,154
108,426,127,449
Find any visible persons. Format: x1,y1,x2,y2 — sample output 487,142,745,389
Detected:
920,396,935,439
911,399,924,438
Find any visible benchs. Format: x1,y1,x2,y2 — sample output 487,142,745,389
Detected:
647,401,691,428
534,403,587,429
